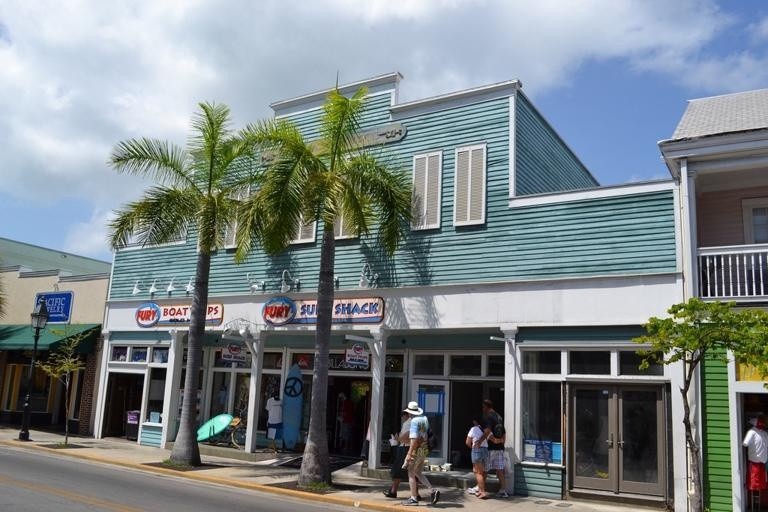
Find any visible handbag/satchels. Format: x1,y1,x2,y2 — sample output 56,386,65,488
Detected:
428,428,437,449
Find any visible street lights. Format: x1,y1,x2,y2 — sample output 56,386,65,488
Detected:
17,295,50,441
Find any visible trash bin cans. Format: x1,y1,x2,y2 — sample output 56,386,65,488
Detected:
127,411,139,441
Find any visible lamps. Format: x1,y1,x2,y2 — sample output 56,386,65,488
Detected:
358,262,378,289
131,277,194,294
280,270,298,293
247,272,265,293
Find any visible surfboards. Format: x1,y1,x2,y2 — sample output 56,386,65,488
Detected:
197,414,233,440
282,364,303,450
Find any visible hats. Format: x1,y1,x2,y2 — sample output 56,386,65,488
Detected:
403,401,423,415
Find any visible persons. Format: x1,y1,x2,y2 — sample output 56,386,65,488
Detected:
265,387,284,455
466,418,506,499
467,399,508,498
743,411,768,491
383,412,421,501
400,401,440,506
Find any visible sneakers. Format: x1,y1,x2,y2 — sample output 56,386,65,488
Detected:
384,489,440,505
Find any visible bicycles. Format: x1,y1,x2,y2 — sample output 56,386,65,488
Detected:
203,396,246,450
